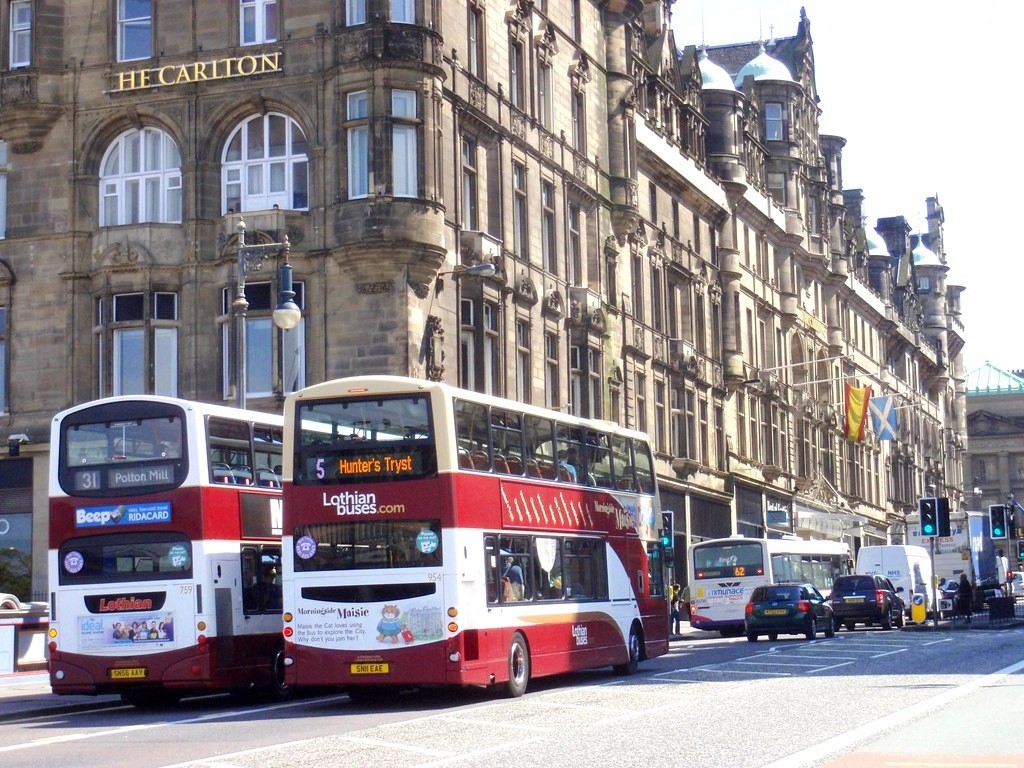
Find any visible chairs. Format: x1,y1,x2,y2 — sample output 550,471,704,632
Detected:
458,446,644,494
211,463,280,487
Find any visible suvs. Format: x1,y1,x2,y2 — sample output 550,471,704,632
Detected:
829,574,906,633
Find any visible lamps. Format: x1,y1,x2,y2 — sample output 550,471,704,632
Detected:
439,264,496,277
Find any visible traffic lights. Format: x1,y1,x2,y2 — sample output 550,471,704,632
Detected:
661,511,673,550
1017,540,1024,558
1006,571,1012,582
918,497,939,537
989,504,1008,539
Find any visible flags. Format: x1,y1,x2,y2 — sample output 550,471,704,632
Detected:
842,382,872,443
869,395,898,441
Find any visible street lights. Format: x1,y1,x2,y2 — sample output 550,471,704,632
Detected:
234,234,301,409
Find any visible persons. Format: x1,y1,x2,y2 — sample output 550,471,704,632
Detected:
681,584,691,622
252,561,280,613
274,465,282,474
557,448,578,484
669,584,683,636
113,621,167,639
958,574,973,623
623,465,643,493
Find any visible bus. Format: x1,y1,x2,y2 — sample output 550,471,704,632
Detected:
281,374,669,697
43,394,411,708
688,534,856,637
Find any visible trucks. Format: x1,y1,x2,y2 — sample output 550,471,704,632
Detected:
995,556,1014,594
905,511,1003,611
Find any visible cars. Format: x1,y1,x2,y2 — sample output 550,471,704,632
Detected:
938,579,960,598
744,583,834,642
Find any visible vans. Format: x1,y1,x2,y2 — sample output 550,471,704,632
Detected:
856,545,945,621
1011,571,1024,596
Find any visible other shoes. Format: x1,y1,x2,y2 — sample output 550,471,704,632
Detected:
964,619,971,624
675,632,682,636
